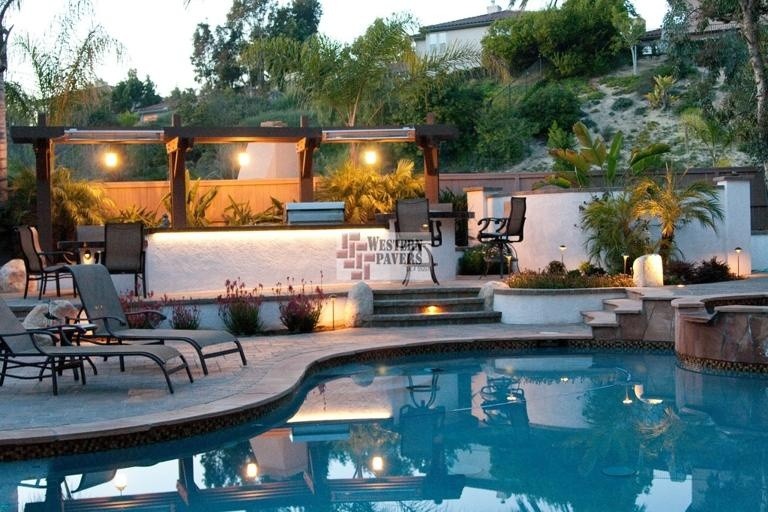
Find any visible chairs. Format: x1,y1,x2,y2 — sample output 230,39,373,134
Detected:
395,196,526,286
72,470,117,493
398,375,530,459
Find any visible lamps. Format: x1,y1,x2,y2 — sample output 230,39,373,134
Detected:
115,472,128,497
364,142,376,164
104,143,116,165
247,457,259,479
237,143,250,168
371,451,384,472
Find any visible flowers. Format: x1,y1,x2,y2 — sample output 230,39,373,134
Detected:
217,271,327,336
